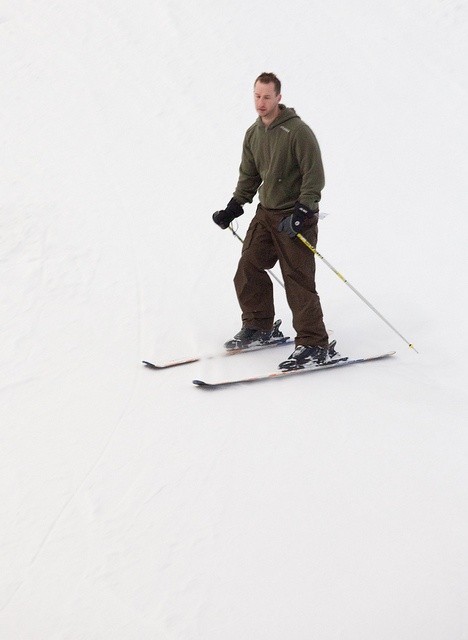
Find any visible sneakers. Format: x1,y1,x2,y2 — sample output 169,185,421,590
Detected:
278,340,341,372
223,319,283,351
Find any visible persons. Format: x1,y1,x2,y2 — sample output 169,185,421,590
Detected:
212,73,340,372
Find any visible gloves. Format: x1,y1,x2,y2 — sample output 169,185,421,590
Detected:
277,201,314,240
212,197,244,230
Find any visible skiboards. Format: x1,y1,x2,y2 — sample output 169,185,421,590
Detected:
142,320,396,386
